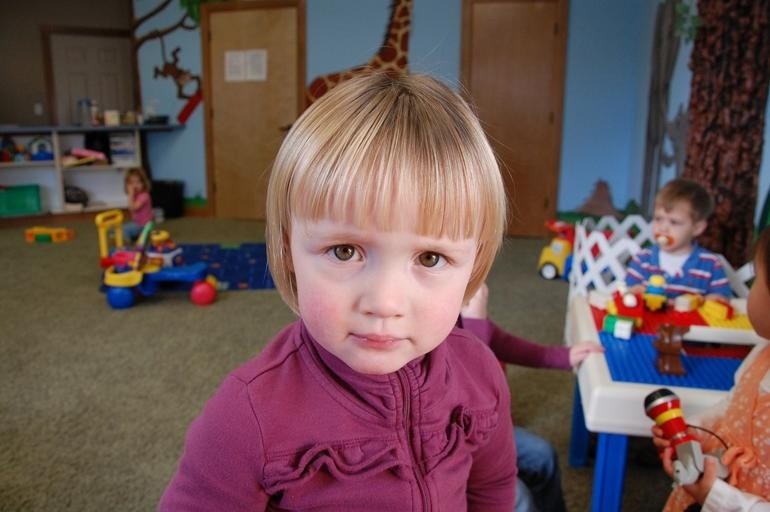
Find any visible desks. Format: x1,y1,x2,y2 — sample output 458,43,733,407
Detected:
565,287,754,510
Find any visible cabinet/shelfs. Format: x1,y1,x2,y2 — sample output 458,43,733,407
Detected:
0,123,144,219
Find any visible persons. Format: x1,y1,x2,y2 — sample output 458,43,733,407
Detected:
156,71,518,512
652,225,770,512
109,167,156,242
455,283,606,512
624,179,733,297
